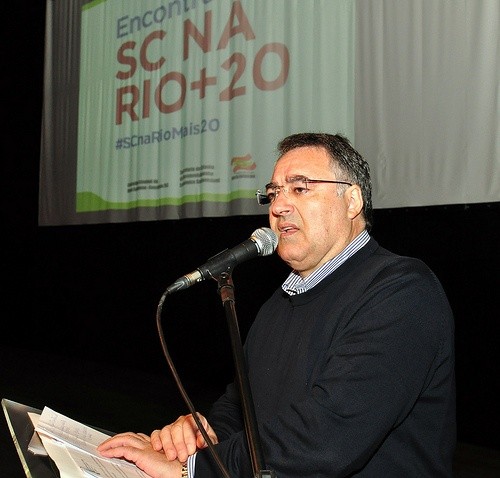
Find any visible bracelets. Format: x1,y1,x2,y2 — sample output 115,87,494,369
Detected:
182,462,187,478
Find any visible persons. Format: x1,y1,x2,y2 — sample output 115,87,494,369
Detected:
96,133,455,478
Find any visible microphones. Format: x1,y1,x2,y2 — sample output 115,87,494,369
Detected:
167,227,278,293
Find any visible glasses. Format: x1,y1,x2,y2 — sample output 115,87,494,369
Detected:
256,174,352,207
